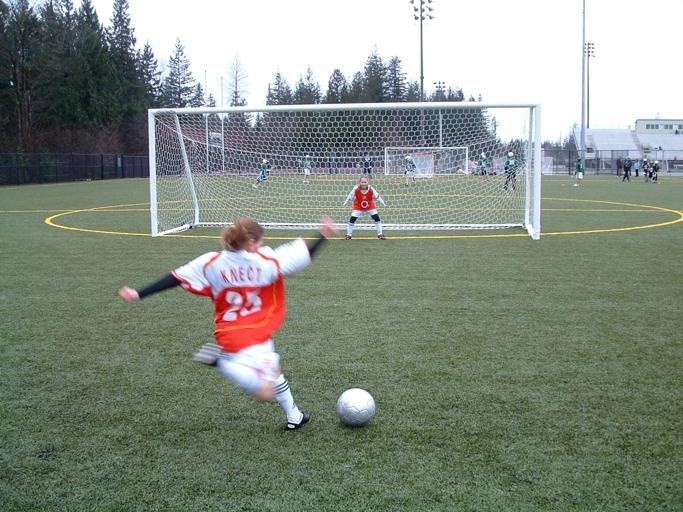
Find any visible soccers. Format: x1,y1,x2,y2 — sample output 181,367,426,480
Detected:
337,388,375,426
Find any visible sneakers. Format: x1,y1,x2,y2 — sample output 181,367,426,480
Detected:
288,412,310,429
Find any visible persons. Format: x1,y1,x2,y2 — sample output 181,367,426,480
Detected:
469,149,496,176
360,153,373,180
570,157,583,188
113,212,340,432
613,155,662,185
249,157,272,189
403,155,417,186
301,154,313,185
342,176,389,243
503,150,517,192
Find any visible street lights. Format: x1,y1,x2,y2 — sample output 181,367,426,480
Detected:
408,0,437,147
584,42,597,129
